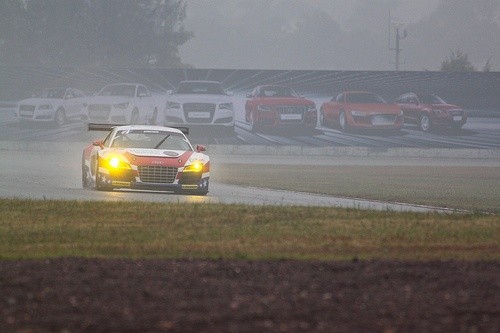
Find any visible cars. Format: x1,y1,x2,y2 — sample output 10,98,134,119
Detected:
162,80,236,134
321,87,406,131
395,90,469,133
89,79,156,129
9,86,88,128
244,85,318,131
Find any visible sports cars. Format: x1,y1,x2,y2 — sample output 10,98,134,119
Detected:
79,123,211,195
